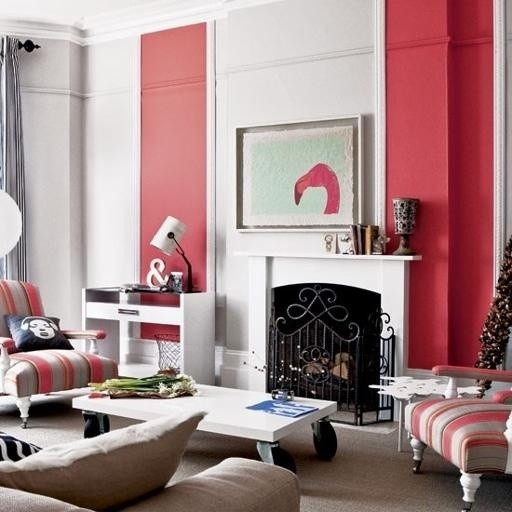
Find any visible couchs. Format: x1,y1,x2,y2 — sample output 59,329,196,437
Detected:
0,454,299,512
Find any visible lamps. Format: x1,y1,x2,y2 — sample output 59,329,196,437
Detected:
391,195,420,256
150,215,201,292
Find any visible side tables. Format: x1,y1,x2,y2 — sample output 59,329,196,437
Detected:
368,372,483,454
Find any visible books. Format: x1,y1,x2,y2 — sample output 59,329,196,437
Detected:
350,225,380,254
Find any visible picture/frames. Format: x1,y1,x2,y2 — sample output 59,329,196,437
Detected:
235,114,364,233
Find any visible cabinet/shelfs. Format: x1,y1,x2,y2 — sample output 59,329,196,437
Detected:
81,283,215,384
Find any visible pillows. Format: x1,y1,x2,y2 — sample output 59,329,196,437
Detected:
3,313,76,351
0,411,208,509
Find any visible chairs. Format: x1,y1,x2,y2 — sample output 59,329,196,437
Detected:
0,279,119,430
405,365,512,512
